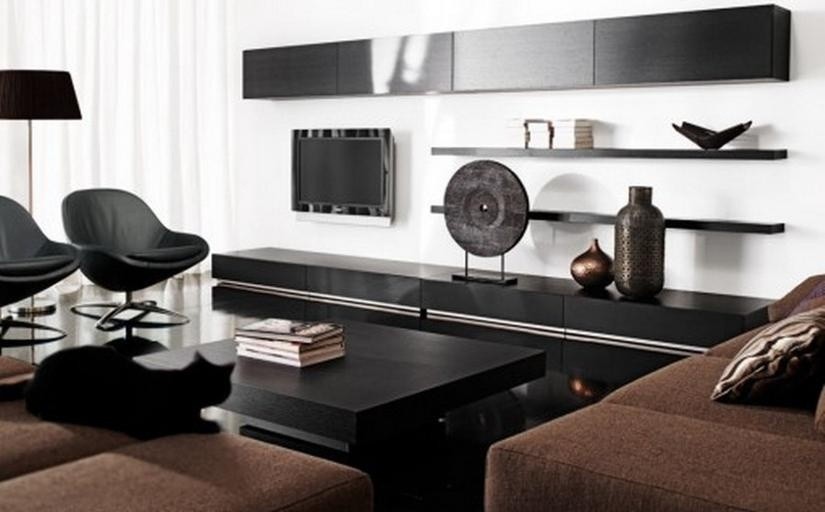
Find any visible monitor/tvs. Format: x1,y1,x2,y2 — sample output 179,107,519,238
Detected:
291,128,394,228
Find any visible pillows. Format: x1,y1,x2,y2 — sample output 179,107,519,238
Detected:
711,306,825,403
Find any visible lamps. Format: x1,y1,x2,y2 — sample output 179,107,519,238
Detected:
0,69,83,314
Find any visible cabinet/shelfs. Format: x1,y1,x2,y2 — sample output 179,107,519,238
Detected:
451,4,792,93
241,31,453,101
211,245,781,383
430,147,788,236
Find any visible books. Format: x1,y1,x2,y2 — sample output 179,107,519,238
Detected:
235,318,346,370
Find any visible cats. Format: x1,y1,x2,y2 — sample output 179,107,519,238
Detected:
23,344,236,441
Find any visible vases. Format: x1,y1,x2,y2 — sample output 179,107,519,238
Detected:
615,186,665,299
570,237,615,291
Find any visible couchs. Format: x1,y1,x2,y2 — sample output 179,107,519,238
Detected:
483,274,825,511
1,356,378,511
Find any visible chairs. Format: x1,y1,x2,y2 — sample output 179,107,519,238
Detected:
0,195,81,344
63,190,208,327
